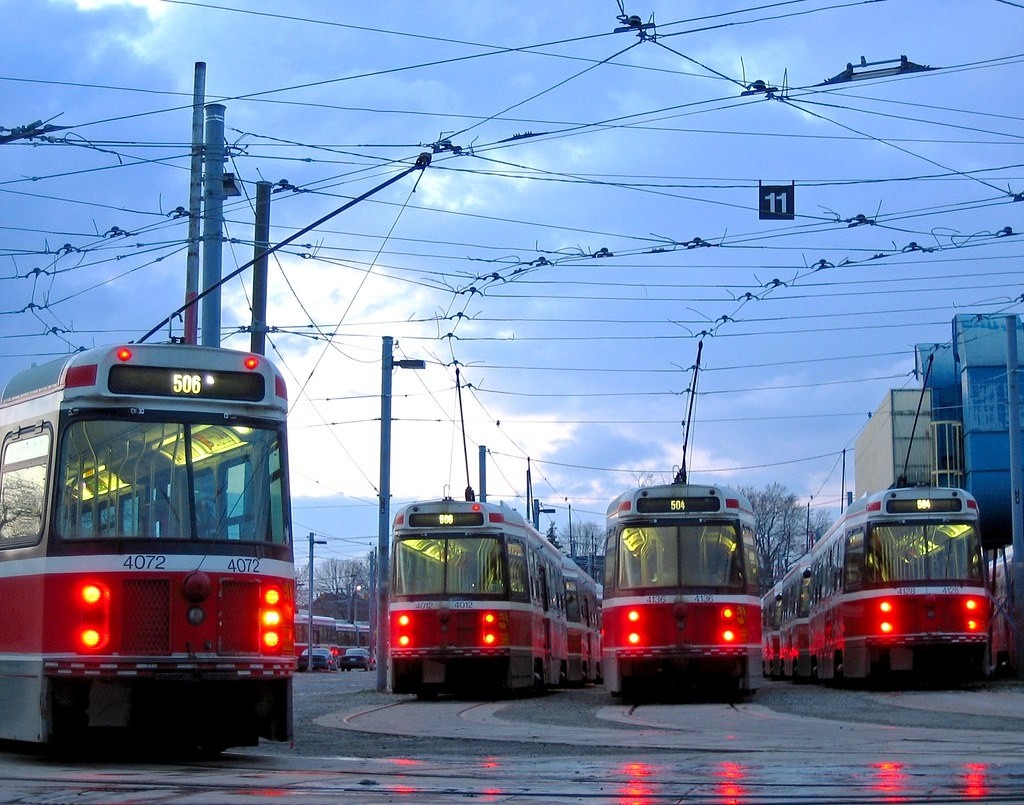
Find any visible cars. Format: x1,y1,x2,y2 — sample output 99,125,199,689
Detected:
298,647,338,673
339,648,377,672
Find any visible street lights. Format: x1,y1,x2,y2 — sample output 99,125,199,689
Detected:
308,532,327,674
376,335,426,695
534,498,555,532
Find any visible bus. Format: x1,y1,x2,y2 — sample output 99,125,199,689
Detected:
0,151,435,764
388,367,602,698
293,613,376,663
764,353,997,685
599,341,763,703
